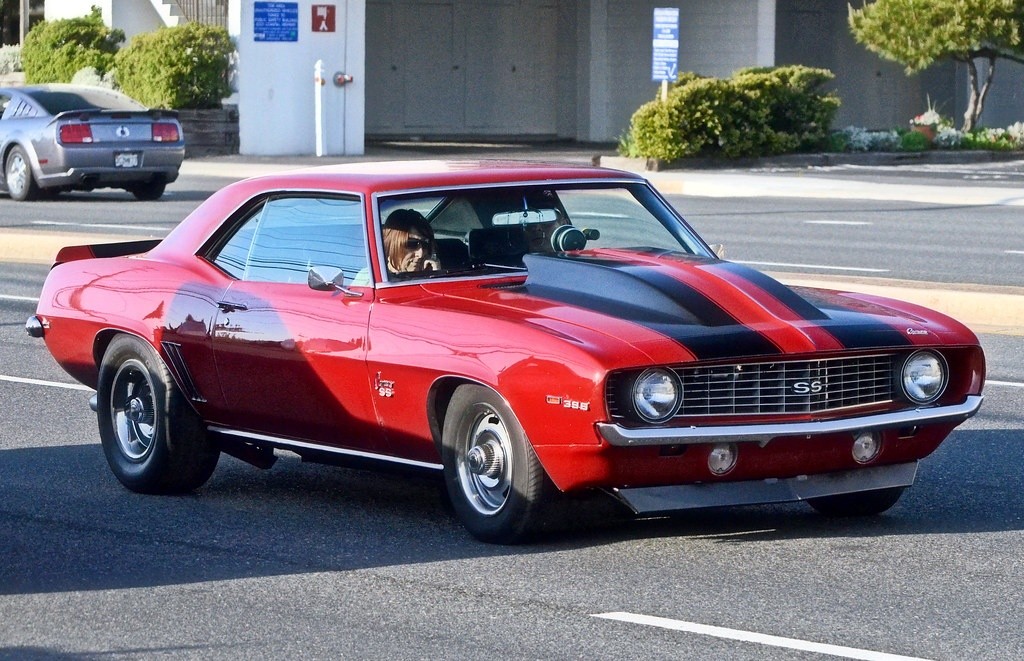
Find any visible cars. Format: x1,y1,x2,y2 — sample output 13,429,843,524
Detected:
0,82,186,202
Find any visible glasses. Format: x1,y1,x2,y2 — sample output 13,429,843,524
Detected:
403,238,433,249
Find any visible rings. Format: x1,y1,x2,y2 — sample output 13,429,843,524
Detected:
431,252,437,260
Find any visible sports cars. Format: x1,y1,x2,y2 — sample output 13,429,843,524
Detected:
21,158,987,546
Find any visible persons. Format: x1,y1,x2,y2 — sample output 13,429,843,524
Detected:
351,208,442,286
502,200,570,273
